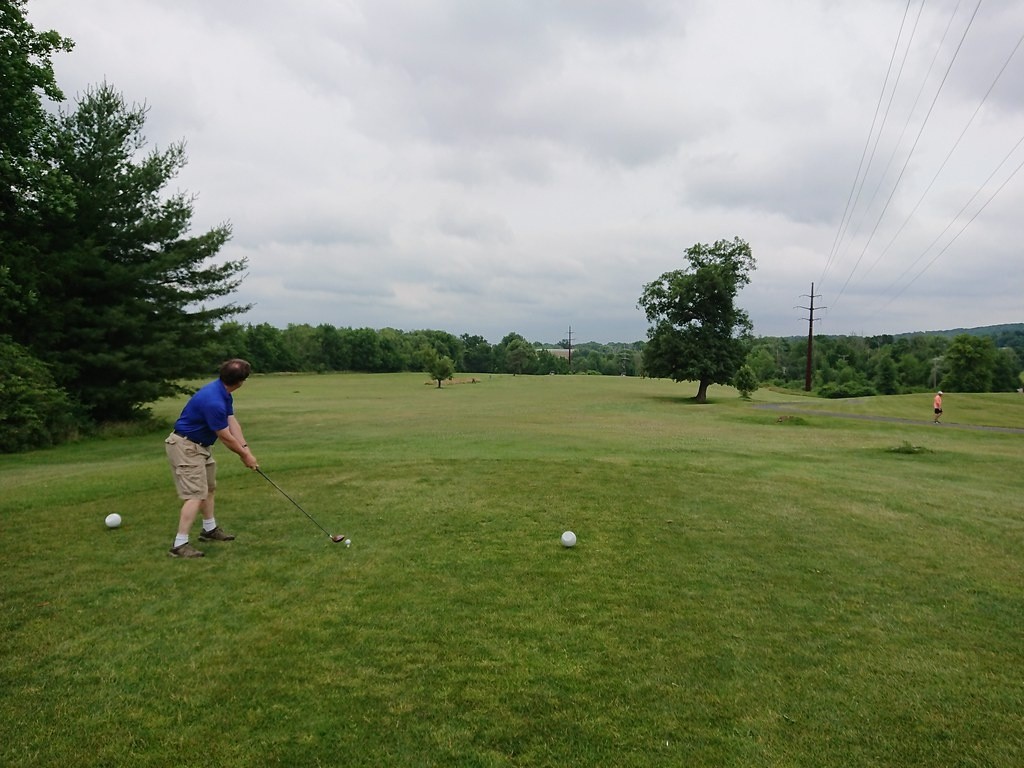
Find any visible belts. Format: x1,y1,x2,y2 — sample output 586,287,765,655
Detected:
173,431,210,448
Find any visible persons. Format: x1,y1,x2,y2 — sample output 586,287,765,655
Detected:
934,391,944,424
162,359,260,559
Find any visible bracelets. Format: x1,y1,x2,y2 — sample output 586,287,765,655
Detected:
242,443,249,449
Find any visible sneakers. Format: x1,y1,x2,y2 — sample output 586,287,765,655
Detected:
169,542,205,559
199,526,235,541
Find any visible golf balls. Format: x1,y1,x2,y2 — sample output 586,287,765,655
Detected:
346,539,351,546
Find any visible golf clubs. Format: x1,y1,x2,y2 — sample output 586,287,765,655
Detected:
256,467,346,543
